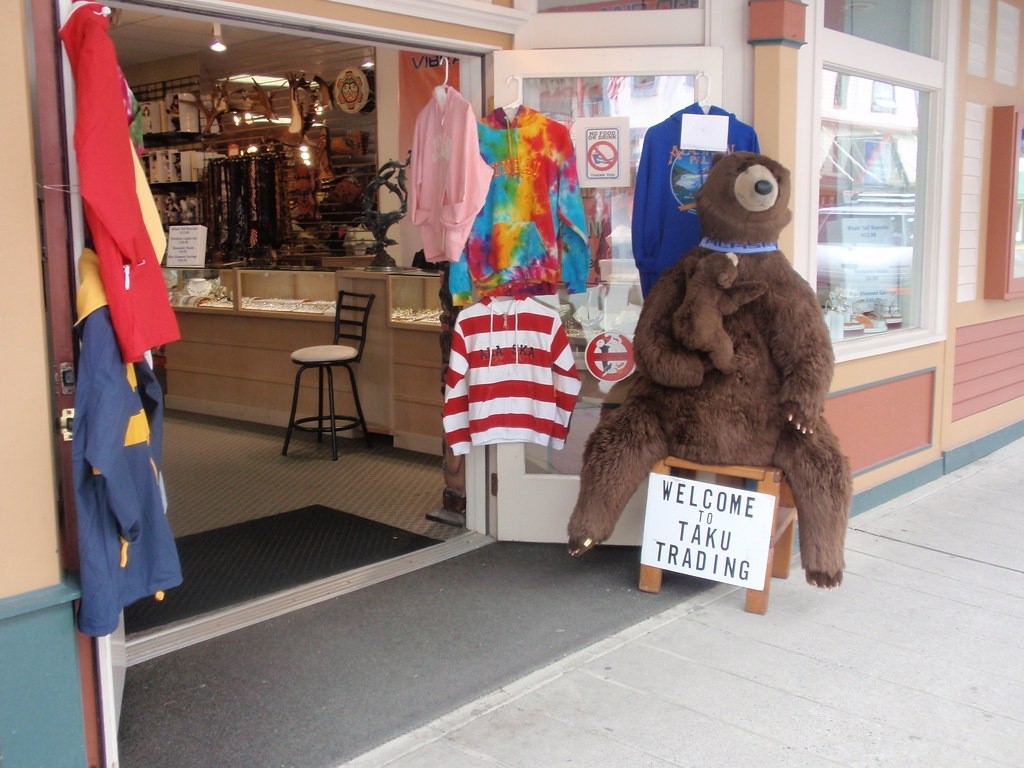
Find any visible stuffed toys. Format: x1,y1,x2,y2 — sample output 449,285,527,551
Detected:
567,152,852,590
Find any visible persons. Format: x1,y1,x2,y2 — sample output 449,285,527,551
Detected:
170,95,181,131
143,107,151,129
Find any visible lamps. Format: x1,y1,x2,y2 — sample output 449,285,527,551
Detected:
210,23,227,52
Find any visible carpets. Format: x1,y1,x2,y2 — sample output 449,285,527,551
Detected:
123,504,445,635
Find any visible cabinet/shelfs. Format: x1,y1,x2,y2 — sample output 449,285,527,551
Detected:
129,75,206,232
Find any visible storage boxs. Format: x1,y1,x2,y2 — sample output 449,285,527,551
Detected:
141,149,205,183
138,93,199,132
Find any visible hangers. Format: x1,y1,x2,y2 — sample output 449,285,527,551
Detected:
695,74,712,108
503,76,522,109
440,57,450,89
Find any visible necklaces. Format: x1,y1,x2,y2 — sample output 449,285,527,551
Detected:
187,283,210,295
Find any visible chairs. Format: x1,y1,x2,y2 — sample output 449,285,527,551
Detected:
281,290,375,461
